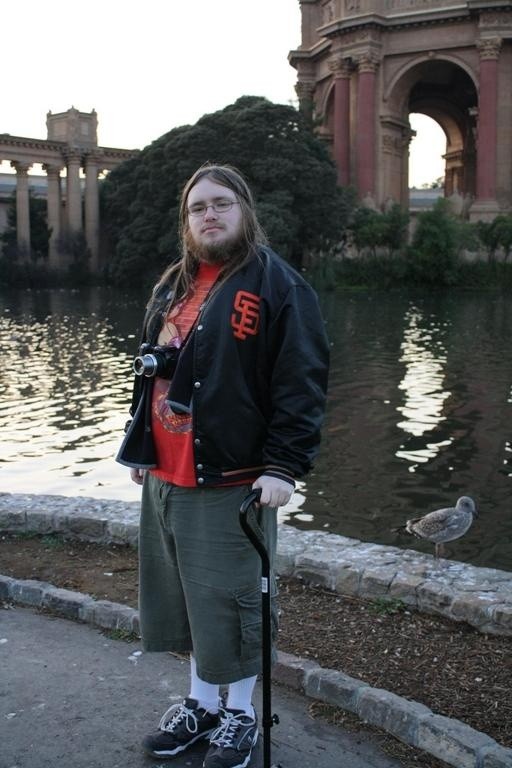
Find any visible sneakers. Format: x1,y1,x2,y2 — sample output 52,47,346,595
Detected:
141,695,225,760
202,699,258,767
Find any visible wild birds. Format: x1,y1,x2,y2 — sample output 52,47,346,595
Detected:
389,495,483,561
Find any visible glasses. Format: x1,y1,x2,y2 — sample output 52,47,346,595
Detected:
187,199,240,218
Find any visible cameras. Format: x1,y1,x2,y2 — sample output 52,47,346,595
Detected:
134,343,179,381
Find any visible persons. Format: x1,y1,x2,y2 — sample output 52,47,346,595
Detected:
115,161,334,768
